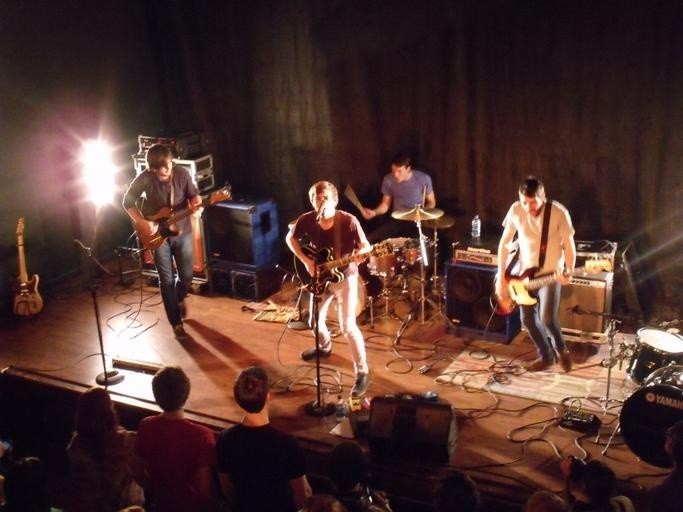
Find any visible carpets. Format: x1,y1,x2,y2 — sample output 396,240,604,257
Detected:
435,328,637,417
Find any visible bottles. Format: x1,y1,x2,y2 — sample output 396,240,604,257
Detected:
336,392,349,422
471,215,482,248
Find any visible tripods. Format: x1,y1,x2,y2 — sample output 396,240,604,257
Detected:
359,273,408,330
393,220,455,346
566,319,625,445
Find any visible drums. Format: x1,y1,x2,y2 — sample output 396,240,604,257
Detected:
619,368,683,470
368,238,402,279
395,234,422,264
627,324,683,384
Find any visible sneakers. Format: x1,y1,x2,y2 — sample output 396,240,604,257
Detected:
177,300,187,317
173,322,188,340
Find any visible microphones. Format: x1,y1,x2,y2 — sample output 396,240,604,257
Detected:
315,199,329,221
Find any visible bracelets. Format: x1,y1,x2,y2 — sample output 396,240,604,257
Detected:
372,209,377,217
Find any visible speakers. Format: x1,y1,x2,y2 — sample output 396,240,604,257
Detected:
443,257,522,344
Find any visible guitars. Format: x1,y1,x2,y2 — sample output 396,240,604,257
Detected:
133,178,233,249
490,261,605,314
294,239,396,295
9,210,45,319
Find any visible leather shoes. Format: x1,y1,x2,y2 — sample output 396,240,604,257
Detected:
303,349,331,362
351,371,372,398
524,355,557,371
557,349,572,374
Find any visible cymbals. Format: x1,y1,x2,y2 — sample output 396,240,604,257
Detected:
423,215,455,231
393,205,446,223
287,219,297,230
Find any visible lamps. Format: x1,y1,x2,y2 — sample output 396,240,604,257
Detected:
55,134,123,385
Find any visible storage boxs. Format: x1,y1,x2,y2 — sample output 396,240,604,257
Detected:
134,154,216,194
202,194,281,267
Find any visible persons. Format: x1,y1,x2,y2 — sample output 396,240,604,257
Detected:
359,152,437,301
528,419,683,511
285,180,373,400
434,471,480,510
123,145,202,340
494,176,576,373
1,368,390,510
